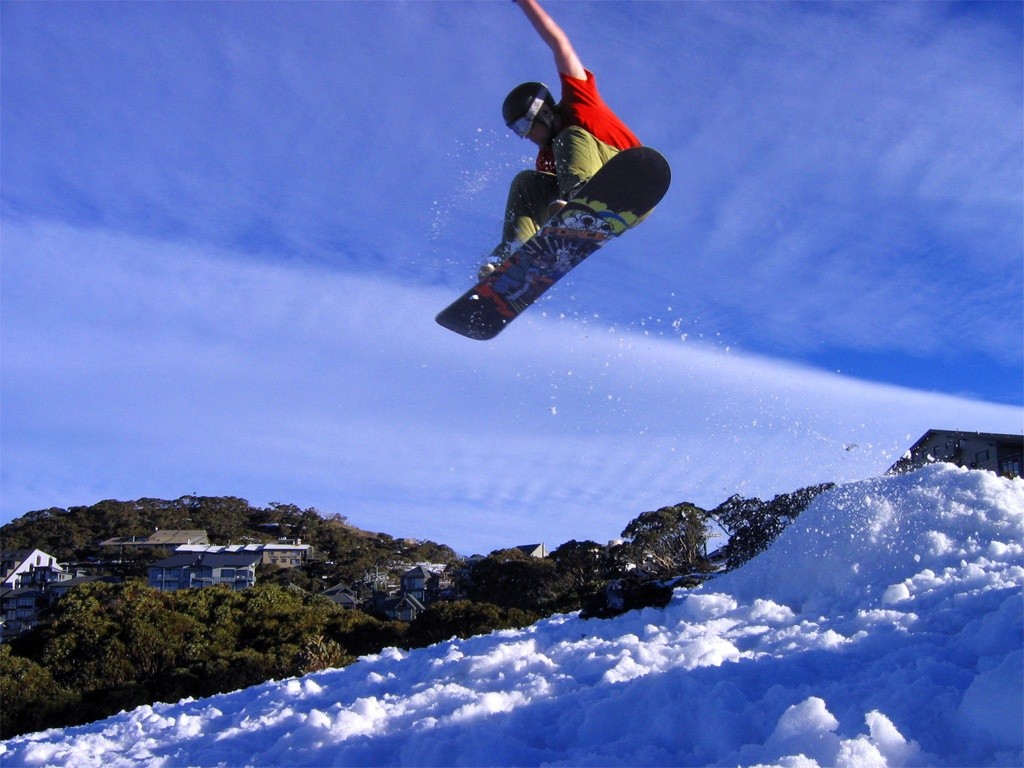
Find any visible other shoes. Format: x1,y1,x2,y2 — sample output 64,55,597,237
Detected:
548,199,567,217
479,263,495,280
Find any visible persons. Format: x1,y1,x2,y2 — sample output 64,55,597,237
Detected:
478,0,654,278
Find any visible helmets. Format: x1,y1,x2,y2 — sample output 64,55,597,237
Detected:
503,82,556,126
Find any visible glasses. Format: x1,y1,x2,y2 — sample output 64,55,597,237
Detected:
507,116,533,137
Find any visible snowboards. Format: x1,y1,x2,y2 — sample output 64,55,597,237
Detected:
433,145,673,343
